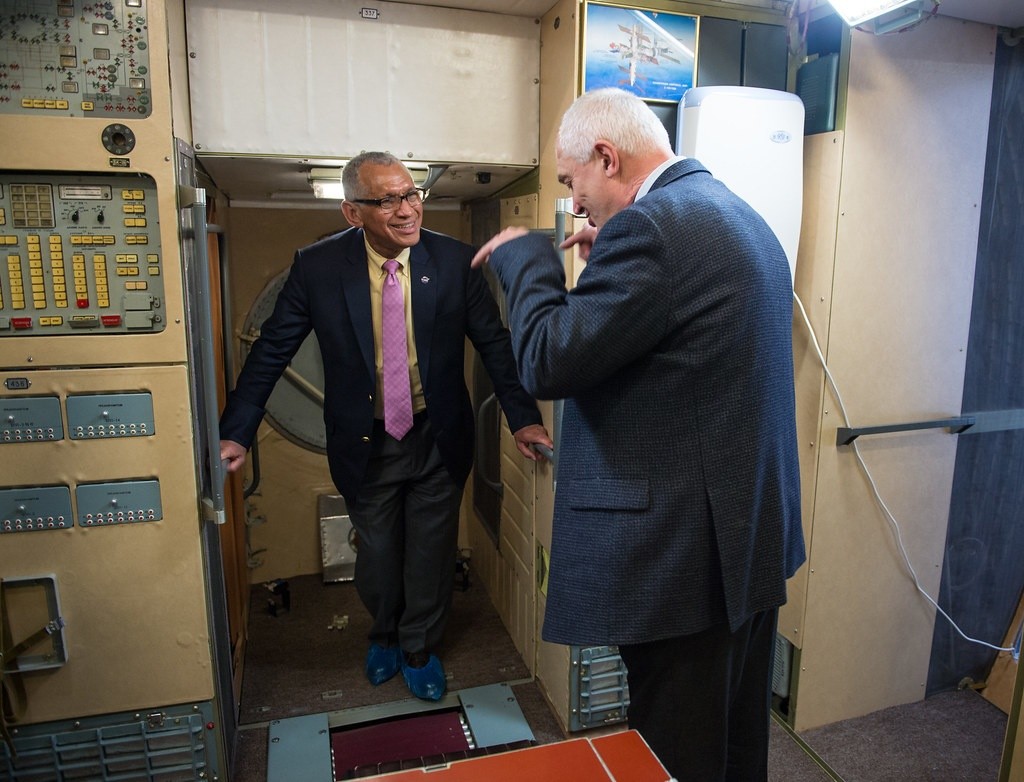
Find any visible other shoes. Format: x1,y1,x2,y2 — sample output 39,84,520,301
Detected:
366,642,402,683
403,651,445,699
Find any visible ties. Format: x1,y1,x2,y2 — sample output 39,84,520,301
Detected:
381,260,414,441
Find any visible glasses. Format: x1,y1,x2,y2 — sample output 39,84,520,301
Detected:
352,188,426,210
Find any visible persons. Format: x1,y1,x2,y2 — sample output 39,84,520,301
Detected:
207,151,554,701
470,86,806,782
